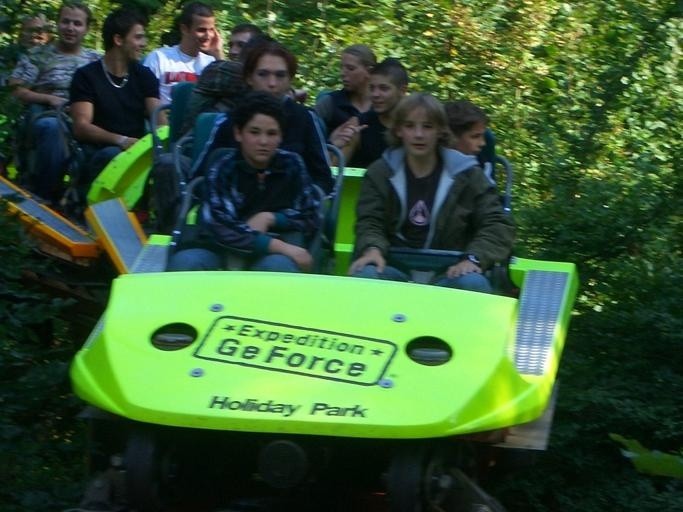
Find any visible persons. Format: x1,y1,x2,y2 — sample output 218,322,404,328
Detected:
1,0,521,295
